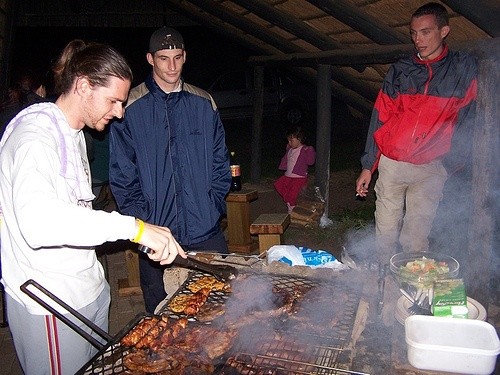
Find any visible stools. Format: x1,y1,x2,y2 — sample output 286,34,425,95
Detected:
251,214,290,255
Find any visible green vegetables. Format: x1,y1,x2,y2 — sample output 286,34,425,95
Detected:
399,257,444,285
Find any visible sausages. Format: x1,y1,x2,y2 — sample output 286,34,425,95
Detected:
224,338,318,375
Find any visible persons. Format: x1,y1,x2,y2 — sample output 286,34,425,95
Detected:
0,40,185,375
276,125,317,215
355,2,479,268
104,26,230,319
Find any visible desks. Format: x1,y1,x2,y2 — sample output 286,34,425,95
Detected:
225,190,260,252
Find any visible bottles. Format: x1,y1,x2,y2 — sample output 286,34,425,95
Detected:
230,151,242,192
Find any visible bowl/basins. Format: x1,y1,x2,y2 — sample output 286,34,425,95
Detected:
404,315,500,375
389,250,461,294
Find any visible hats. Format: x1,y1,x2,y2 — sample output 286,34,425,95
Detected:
149,26,184,53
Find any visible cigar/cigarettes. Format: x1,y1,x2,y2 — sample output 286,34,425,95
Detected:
356,193,359,196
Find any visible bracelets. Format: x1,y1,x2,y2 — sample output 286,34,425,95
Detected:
129,217,144,242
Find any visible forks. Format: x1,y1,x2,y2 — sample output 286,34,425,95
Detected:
399,286,434,315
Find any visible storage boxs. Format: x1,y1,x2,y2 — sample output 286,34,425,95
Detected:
434,279,468,318
405,315,500,375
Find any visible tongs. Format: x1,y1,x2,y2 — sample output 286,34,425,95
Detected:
138,244,238,283
